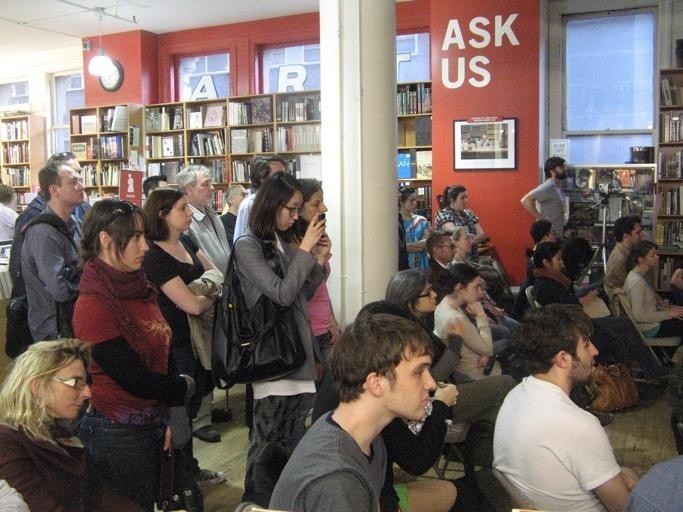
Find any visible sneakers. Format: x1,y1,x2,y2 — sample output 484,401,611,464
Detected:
194,469,227,486
585,408,615,427
211,408,233,422
194,425,221,443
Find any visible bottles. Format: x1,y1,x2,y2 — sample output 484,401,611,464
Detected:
588,168,596,189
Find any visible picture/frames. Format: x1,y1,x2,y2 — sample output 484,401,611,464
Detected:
452,116,517,171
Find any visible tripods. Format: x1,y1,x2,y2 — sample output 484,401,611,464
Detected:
577,214,615,285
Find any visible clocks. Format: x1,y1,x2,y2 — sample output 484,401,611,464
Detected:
97,59,124,93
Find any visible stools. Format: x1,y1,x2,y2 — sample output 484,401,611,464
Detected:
430,421,473,481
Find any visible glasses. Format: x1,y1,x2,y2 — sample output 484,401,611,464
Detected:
418,283,433,297
49,375,86,390
436,244,457,251
279,200,306,218
98,199,137,229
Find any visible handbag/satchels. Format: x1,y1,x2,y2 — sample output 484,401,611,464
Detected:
154,440,204,512
5,294,34,358
587,359,639,412
210,235,306,390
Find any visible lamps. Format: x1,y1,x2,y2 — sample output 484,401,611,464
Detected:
88,14,113,75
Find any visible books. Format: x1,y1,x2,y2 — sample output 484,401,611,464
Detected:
1,120,27,213
72,92,324,211
397,83,431,220
658,77,683,286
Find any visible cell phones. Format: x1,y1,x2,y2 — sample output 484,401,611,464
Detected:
317,213,327,229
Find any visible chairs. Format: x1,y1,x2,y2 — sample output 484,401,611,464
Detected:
523,284,540,310
614,295,680,386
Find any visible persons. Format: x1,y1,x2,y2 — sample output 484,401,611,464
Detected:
261,158,682,511
1,152,342,512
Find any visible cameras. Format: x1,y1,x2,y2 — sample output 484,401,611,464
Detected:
598,181,613,196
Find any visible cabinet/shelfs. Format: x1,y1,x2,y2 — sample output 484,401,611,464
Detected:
144,88,321,216
654,68,682,295
395,80,431,183
560,162,655,287
0,114,46,214
68,103,142,207
